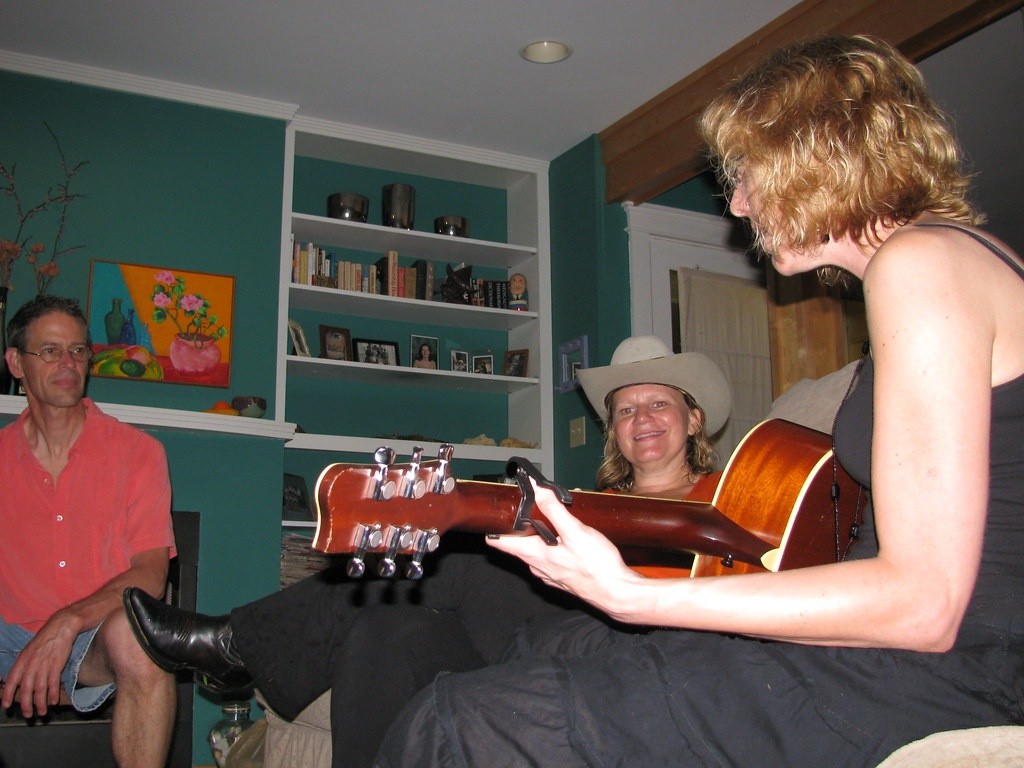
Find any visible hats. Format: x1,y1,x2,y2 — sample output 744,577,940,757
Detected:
573,333,733,438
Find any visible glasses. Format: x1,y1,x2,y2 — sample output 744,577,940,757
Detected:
18,346,93,364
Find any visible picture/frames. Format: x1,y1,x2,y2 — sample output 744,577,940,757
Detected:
451,349,468,374
472,354,494,375
319,324,352,363
503,349,529,378
351,333,401,367
410,333,441,373
287,318,313,358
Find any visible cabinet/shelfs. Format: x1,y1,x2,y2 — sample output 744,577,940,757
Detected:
275,115,556,526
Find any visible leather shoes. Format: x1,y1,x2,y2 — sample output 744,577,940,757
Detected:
122,584,256,698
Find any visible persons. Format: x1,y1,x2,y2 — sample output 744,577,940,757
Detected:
124,334,736,768
508,273,530,311
0,296,179,768
413,343,437,369
365,344,389,365
376,27,1024,768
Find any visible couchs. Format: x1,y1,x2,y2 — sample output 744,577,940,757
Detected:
255,582,1024,768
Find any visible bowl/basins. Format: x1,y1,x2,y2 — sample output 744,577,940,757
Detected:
232,396,266,418
327,194,371,223
435,217,469,236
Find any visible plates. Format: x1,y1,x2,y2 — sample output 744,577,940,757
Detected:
200,408,240,416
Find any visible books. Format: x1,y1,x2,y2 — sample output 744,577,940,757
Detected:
469,276,512,309
290,233,435,301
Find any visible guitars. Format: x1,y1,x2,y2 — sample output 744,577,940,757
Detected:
309,416,864,581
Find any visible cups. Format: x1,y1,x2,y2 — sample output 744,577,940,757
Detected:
383,183,415,229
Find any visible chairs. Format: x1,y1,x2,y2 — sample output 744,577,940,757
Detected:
0,512,201,768
